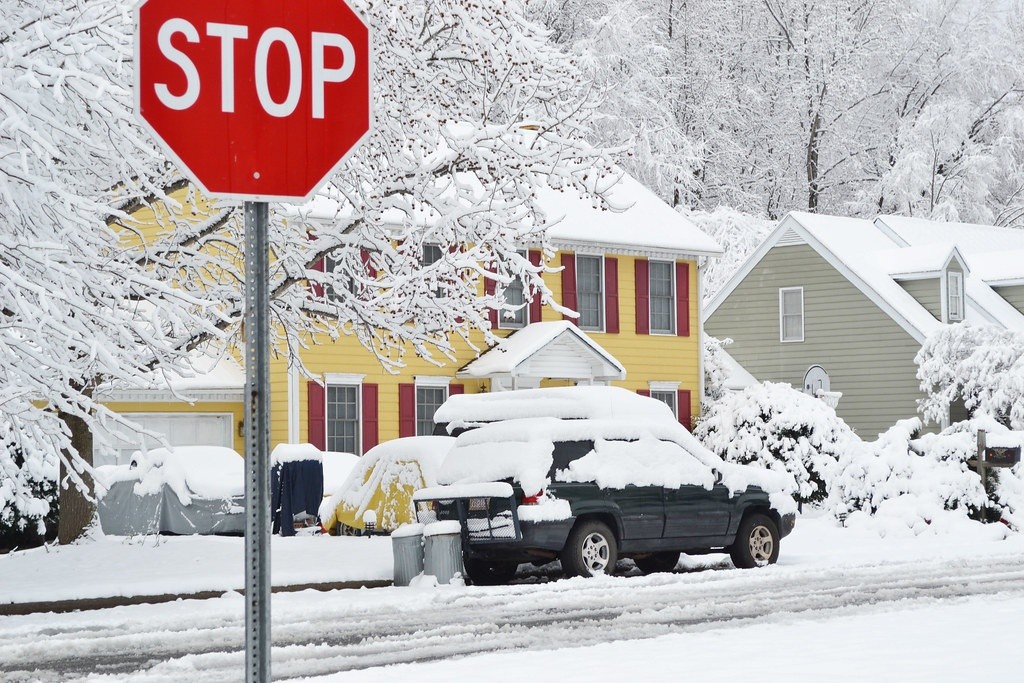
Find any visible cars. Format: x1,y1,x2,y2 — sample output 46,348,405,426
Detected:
270,433,458,539
97,446,246,539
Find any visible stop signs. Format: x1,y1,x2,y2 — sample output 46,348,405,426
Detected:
130,1,372,202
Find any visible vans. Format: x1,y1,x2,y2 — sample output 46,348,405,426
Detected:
434,394,590,506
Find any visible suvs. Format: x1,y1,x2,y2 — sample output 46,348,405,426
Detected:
436,429,795,588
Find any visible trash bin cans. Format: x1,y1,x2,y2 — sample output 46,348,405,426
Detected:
392,534,422,587
425,532,463,588
